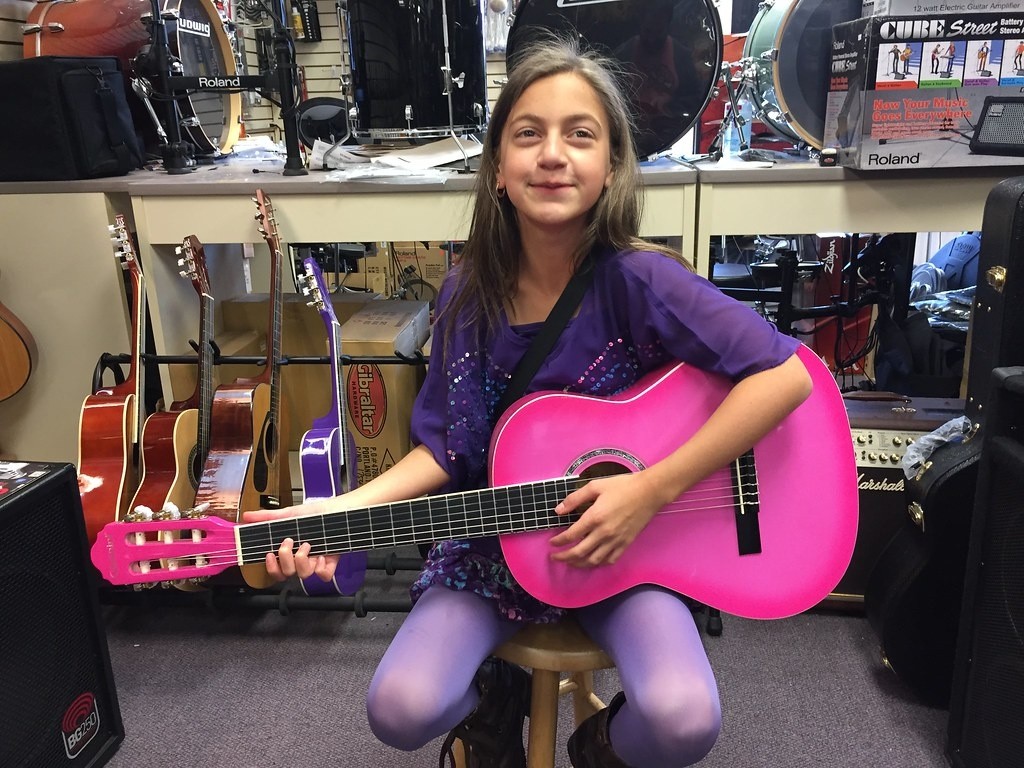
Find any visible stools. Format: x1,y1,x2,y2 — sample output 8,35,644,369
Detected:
453,615,617,768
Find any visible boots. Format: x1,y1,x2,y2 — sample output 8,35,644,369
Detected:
567,691,631,768
439,656,532,768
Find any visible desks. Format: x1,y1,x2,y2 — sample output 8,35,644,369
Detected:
126,133,700,507
679,152,1024,400
0,143,248,477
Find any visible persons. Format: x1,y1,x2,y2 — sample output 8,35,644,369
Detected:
611,0,704,156
243,42,813,768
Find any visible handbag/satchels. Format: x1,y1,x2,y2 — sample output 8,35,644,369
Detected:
0,55,148,182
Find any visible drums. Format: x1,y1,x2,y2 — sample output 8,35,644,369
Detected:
20,0,243,157
345,0,488,137
742,0,862,152
505,0,724,163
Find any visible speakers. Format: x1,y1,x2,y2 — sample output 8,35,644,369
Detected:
1017,70,1024,76
0,459,126,768
940,72,949,78
808,397,967,611
943,366,1024,768
969,96,1024,155
894,73,905,80
835,80,862,148
981,71,992,77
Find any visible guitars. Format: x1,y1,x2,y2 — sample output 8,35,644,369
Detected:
192,188,292,589
87,333,860,622
124,235,216,592
0,300,40,403
297,256,368,596
76,214,148,548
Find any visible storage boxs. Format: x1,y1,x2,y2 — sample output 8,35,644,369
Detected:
167,242,451,491
822,0,1024,171
